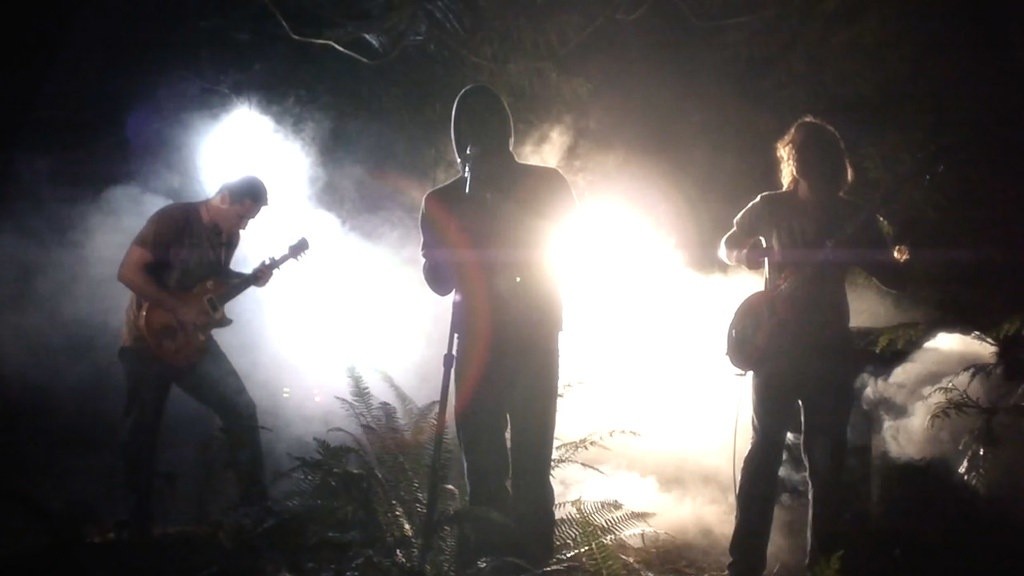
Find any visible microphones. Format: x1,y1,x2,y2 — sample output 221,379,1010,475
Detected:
465,143,481,195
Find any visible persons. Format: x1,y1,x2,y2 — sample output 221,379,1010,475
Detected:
717,119,913,576
117,175,271,510
421,84,576,562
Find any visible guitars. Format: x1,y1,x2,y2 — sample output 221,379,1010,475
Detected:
137,235,310,375
726,143,950,372
